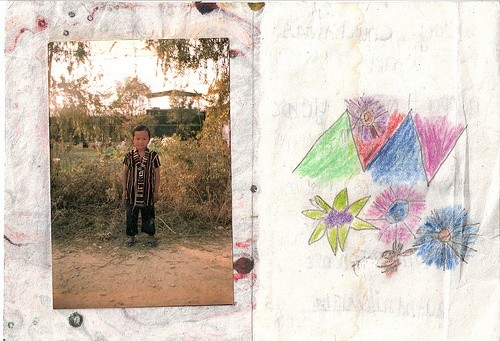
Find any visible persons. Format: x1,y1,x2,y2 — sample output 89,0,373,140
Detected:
121,125,162,248
